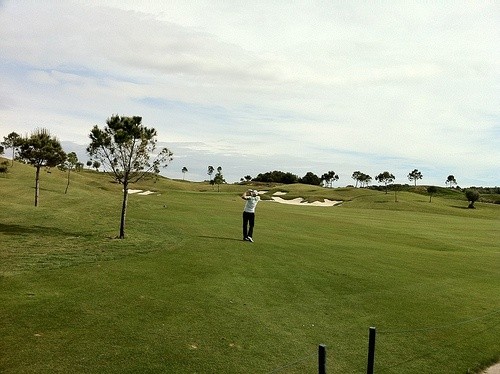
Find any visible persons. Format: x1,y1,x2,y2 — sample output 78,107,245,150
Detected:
241,189,260,243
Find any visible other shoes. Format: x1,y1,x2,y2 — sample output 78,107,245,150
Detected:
244,238,249,240
247,236,254,242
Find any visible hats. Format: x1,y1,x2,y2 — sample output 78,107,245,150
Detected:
251,190,258,196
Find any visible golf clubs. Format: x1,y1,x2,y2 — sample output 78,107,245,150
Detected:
248,189,256,197
